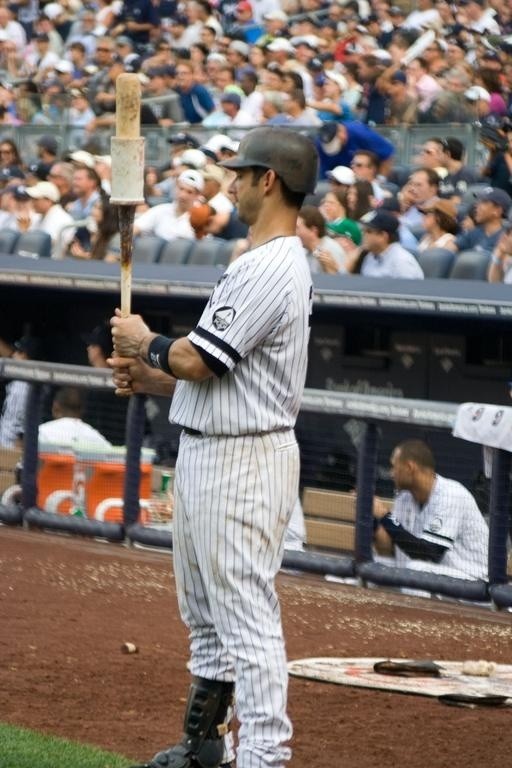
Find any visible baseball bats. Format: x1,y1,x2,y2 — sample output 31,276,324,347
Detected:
110,75,145,396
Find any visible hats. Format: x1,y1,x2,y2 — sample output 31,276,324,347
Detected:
80,325,111,347
1,132,239,240
317,123,511,234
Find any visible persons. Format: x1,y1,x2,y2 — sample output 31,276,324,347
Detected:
1,0,511,282
103,123,322,768
372,438,497,614
3,292,512,445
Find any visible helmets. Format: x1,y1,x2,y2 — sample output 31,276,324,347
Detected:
214,126,317,197
